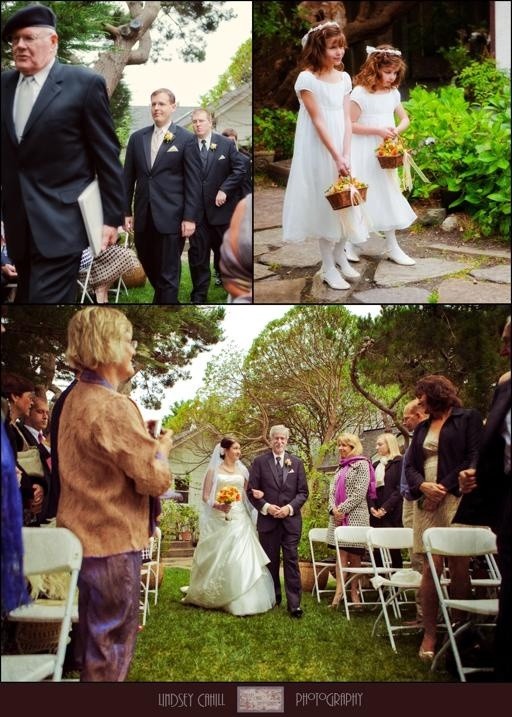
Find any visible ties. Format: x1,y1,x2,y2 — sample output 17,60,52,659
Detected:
151,129,163,168
38,432,46,444
276,457,283,485
15,76,34,141
200,139,209,173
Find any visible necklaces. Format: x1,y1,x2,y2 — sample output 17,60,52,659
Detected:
222,462,237,475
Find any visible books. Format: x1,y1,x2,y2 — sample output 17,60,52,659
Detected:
78,176,106,258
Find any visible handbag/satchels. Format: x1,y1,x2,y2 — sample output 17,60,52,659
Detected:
17,445,44,478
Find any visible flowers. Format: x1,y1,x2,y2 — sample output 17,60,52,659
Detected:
163,129,177,143
284,458,292,467
327,176,366,193
209,143,218,151
378,135,408,157
216,486,241,522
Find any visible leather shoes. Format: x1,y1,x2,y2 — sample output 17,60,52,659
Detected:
288,606,303,618
383,243,416,266
419,633,437,666
351,592,366,612
326,591,343,611
333,246,360,282
320,266,351,290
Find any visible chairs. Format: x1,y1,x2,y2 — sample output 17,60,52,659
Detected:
76,248,96,304
106,223,130,304
304,522,504,682
0,521,87,683
134,535,156,626
139,525,164,607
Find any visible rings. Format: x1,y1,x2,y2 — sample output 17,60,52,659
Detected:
459,487,463,491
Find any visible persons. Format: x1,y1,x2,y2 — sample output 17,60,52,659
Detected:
0,221,20,302
347,41,417,267
458,313,512,683
181,436,264,617
1,4,126,304
222,126,253,208
366,372,486,666
186,104,246,302
281,22,363,291
328,430,376,610
246,424,309,620
118,87,203,302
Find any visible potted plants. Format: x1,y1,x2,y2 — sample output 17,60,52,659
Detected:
140,497,181,591
253,104,296,177
296,466,336,594
176,505,199,542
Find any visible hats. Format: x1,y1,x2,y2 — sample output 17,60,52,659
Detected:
2,5,57,38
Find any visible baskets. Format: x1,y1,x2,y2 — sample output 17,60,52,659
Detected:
325,171,369,210
